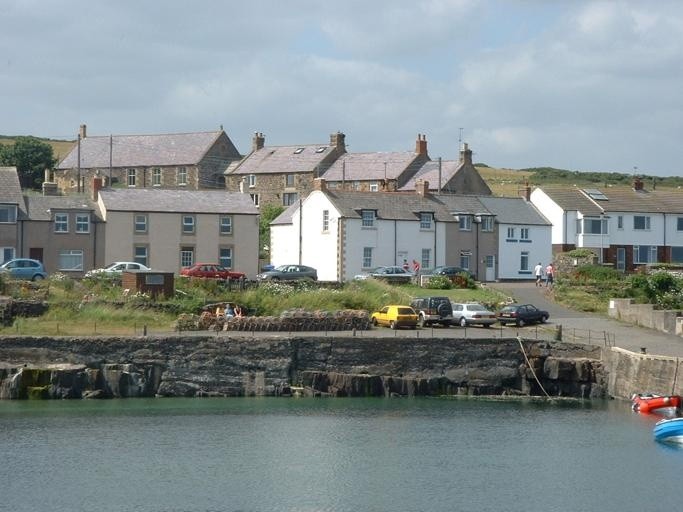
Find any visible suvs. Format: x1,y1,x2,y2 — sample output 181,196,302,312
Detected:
410,297,452,328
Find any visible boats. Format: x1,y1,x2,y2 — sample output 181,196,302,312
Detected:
631,392,682,416
653,417,682,444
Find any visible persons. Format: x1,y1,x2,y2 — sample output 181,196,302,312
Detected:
410,258,421,273
215,304,223,317
232,303,241,316
544,263,554,287
402,259,408,270
533,262,542,287
223,303,233,315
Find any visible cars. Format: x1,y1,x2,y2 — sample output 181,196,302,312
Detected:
431,267,475,282
85,261,151,278
0,258,47,282
496,302,550,327
179,263,245,283
353,266,416,286
370,304,419,330
256,265,318,282
449,302,495,329
